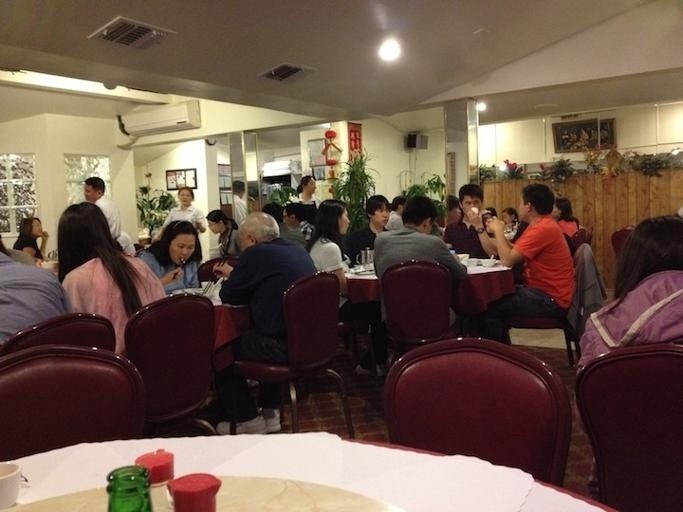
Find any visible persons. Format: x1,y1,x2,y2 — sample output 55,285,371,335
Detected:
82,175,121,242
283,175,321,209
213,212,317,435
572,214,683,488
205,182,579,337
0,187,206,356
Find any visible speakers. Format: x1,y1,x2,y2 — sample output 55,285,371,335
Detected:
418,135,428,149
407,134,418,148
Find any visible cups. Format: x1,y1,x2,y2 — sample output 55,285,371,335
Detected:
0,462,22,509
456,253,495,268
471,207,478,214
360,248,374,270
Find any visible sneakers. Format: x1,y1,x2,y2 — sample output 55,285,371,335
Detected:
356,364,389,382
259,405,283,433
216,408,269,436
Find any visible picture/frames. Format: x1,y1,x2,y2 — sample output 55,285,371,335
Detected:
551,118,617,155
163,169,198,191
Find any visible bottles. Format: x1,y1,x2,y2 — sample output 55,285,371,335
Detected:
166,473,222,512
106,463,152,511
136,447,175,512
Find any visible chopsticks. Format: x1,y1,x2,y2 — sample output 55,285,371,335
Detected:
203,256,228,294
172,261,184,280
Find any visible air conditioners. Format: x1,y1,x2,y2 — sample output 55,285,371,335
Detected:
121,101,200,135
